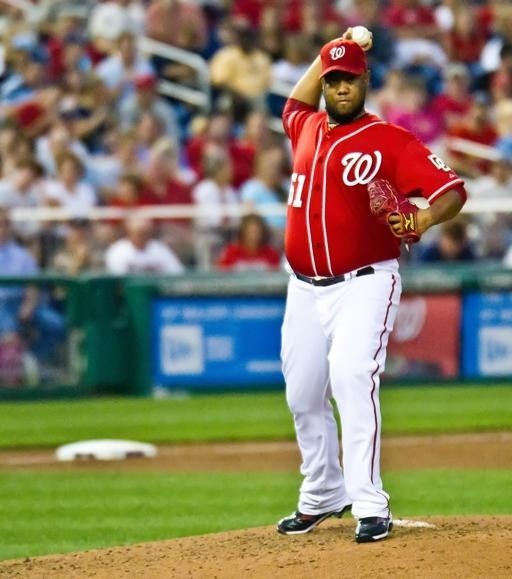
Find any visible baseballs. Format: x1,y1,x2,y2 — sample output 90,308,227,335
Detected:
352,26,370,46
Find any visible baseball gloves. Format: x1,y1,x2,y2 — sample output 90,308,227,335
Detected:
366,179,421,243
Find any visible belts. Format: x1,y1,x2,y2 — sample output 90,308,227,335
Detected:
295,267,374,286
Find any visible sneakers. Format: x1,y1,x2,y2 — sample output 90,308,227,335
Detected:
276,501,351,535
354,508,393,543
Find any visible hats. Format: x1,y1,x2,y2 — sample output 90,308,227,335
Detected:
319,40,368,81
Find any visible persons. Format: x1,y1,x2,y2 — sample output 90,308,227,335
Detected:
1,1,511,273
13,284,75,386
277,26,468,544
